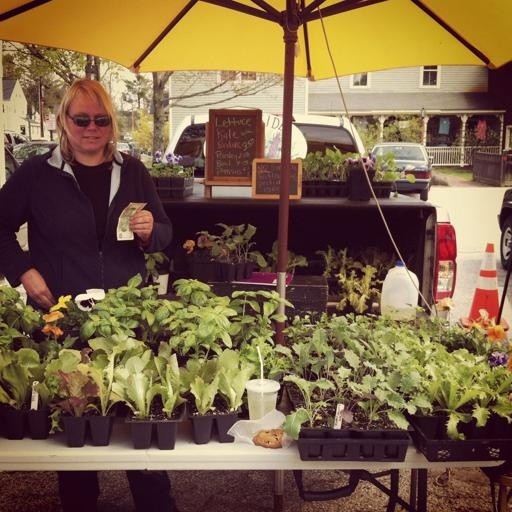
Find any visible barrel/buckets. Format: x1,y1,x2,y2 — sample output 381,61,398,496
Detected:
435,205,458,304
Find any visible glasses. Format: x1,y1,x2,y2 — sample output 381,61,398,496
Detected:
66,111,112,127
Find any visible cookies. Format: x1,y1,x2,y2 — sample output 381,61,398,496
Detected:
252,427,286,449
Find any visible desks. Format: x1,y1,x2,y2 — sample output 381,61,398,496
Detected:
0,361,507,511
160,176,437,319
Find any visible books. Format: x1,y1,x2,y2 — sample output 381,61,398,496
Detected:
232,270,293,288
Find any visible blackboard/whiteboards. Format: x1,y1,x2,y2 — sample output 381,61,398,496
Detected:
205,108,262,186
251,158,302,200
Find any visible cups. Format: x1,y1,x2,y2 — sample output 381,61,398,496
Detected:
245,379,282,422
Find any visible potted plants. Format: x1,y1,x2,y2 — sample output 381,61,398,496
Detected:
299,143,418,201
148,150,195,199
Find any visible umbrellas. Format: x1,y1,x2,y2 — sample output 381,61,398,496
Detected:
0,1,512,353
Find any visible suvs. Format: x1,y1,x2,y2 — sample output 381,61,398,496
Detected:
162,112,209,183
290,112,367,161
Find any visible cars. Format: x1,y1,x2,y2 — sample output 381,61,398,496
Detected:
2,130,145,183
496,185,512,272
368,141,435,201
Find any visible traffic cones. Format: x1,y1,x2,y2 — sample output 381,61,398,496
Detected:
461,239,511,332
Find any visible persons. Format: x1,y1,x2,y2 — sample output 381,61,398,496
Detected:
1,78,179,511
